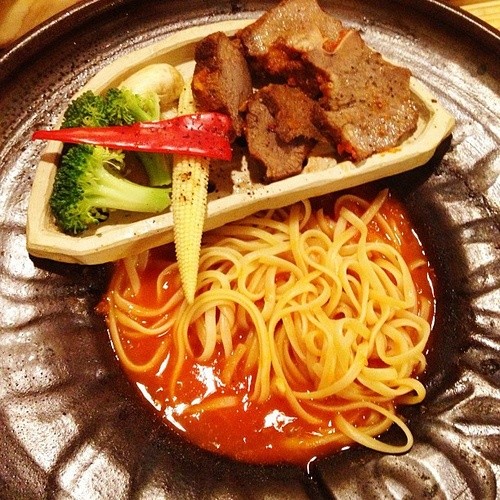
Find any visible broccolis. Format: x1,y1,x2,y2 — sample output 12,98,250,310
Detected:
50,84,174,236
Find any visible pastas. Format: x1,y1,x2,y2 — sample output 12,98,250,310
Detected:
101,188,433,461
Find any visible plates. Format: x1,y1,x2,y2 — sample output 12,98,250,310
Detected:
0,0,499,500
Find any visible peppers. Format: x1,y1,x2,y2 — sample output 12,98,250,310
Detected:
31,112,237,161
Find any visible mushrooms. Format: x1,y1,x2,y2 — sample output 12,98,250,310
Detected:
115,63,184,123
